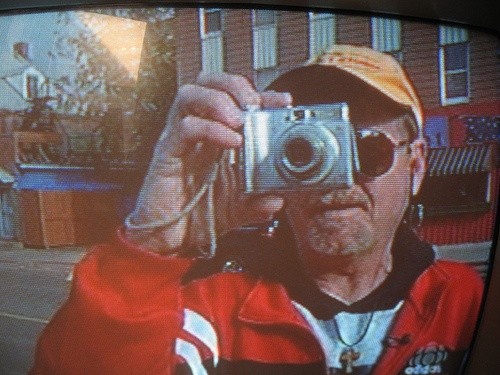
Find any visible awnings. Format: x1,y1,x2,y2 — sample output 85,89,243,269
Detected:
424,147,495,176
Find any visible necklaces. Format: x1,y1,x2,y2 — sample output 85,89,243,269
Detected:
332,291,381,373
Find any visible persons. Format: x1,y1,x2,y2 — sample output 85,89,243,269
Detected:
31,43,482,375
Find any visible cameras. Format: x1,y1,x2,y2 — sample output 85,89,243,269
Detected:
218,99,353,196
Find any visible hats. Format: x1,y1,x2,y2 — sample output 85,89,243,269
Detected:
262,43,423,138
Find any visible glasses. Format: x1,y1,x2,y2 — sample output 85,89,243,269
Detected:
351,127,417,179
